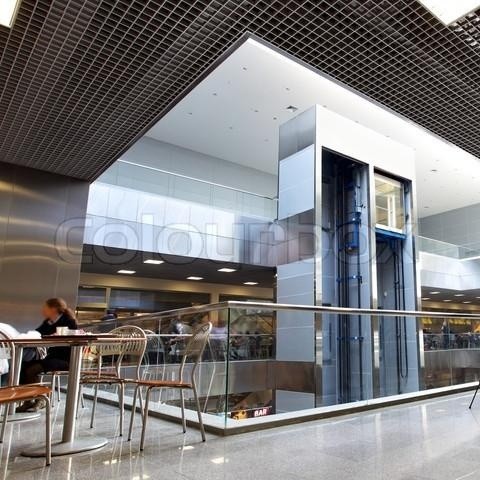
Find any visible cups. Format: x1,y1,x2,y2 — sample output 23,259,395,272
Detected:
56,327,81,335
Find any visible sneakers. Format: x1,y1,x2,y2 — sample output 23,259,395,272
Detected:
16,400,38,414
37,398,47,409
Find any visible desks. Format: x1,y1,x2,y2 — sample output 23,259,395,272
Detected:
1,333,123,457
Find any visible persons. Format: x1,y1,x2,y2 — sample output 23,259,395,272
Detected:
423,321,480,350
97,309,273,361
16,297,79,413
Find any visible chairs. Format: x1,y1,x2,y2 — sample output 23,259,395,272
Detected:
0,330,48,441
77,324,148,435
125,322,215,450
40,370,87,412
0,386,54,480
